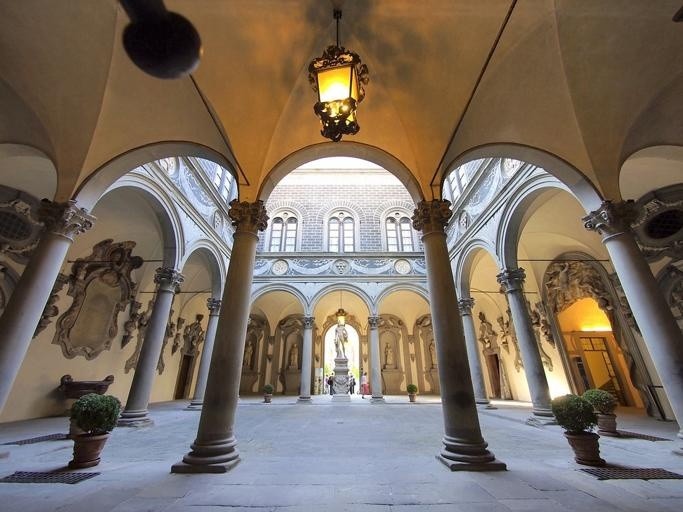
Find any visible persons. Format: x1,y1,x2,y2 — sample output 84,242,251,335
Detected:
361,372,368,399
326,373,334,395
349,375,356,394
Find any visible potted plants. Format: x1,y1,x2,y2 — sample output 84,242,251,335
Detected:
407,384,418,402
583,389,619,437
552,394,606,466
263,384,273,403
66,392,122,467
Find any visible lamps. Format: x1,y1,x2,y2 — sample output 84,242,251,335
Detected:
308,8,370,141
335,289,348,326
